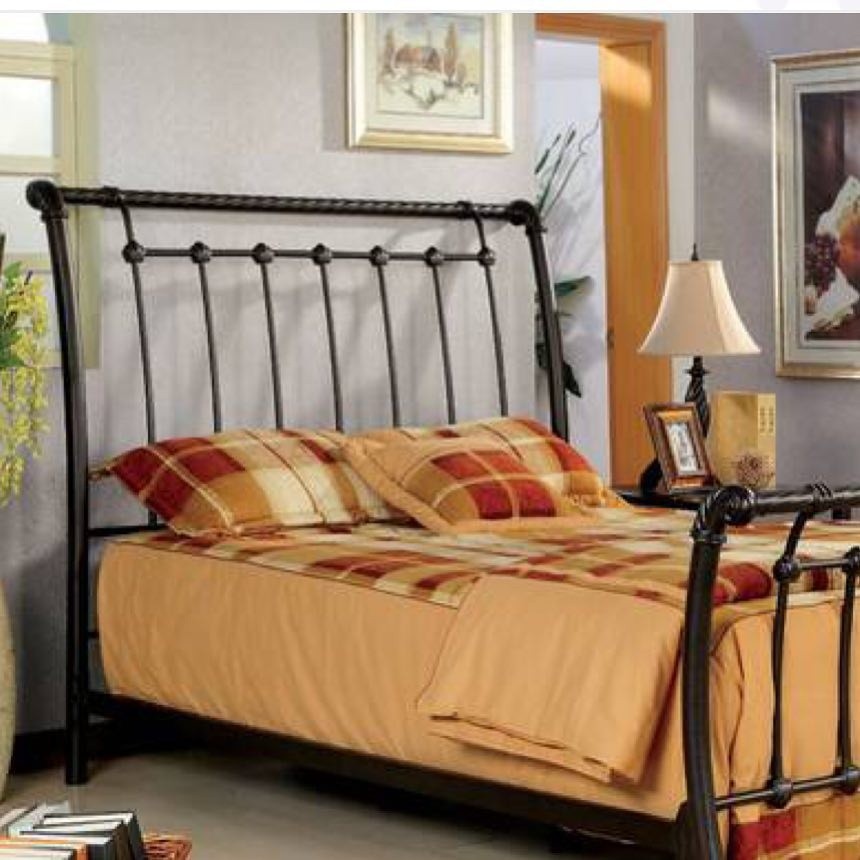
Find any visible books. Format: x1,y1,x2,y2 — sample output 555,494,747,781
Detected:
1,802,146,859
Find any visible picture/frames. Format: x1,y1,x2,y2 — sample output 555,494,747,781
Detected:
346,13,520,154
767,50,860,382
637,399,716,498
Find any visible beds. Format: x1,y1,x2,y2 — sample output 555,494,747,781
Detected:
25,181,860,860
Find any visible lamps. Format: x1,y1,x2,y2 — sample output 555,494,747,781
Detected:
637,241,761,484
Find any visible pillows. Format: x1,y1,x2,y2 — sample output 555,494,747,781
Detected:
102,416,620,547
